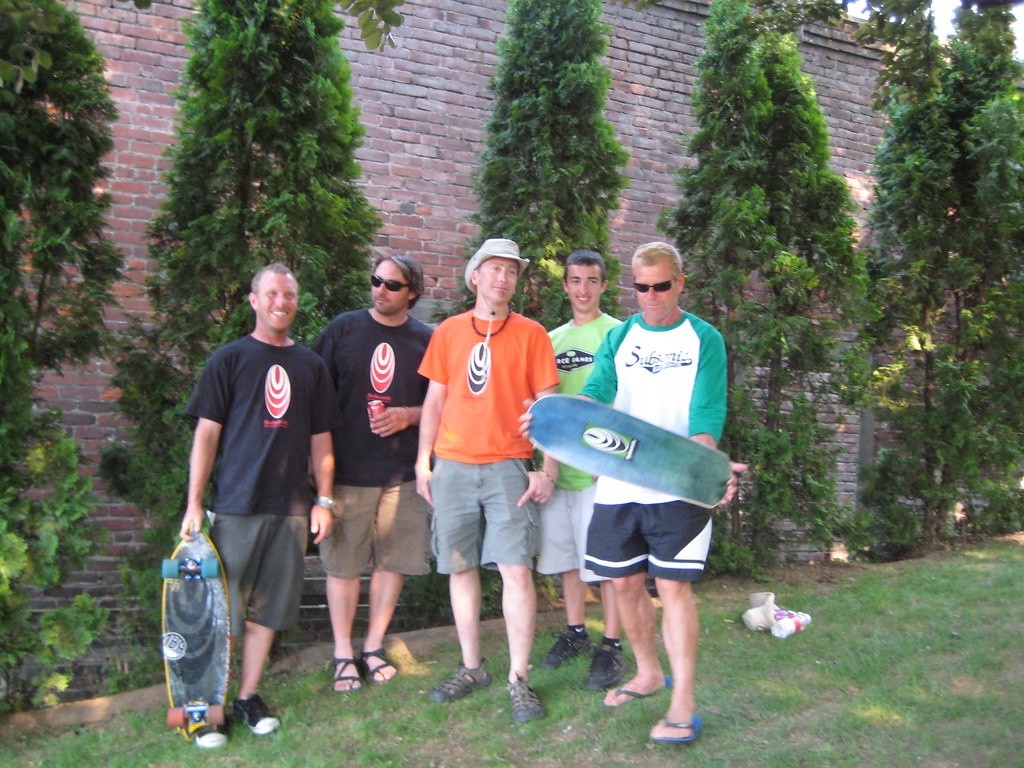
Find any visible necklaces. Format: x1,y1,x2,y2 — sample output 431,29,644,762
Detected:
471,308,511,338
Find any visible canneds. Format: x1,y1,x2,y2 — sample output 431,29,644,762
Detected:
367,399,386,424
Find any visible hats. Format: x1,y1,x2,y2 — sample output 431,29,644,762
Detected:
465,237,531,294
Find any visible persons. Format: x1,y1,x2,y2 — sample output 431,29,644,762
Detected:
179,264,344,749
518,242,749,742
415,238,559,721
531,249,625,690
310,252,435,692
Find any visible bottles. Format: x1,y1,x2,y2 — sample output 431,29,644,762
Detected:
771,613,811,638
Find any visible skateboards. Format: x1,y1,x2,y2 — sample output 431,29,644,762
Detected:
525,393,732,509
161,530,231,742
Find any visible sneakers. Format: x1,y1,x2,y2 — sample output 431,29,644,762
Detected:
232,693,281,735
539,630,591,669
195,724,227,749
585,645,625,690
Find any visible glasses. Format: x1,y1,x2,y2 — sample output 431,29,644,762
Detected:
370,274,410,291
633,279,678,292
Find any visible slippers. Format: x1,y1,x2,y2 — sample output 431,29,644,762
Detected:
654,713,700,742
606,675,673,707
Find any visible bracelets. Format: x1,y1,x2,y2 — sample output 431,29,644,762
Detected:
317,496,335,510
541,470,555,483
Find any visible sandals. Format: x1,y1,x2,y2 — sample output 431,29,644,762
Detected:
333,656,363,693
506,671,545,723
430,656,492,703
360,647,398,684
